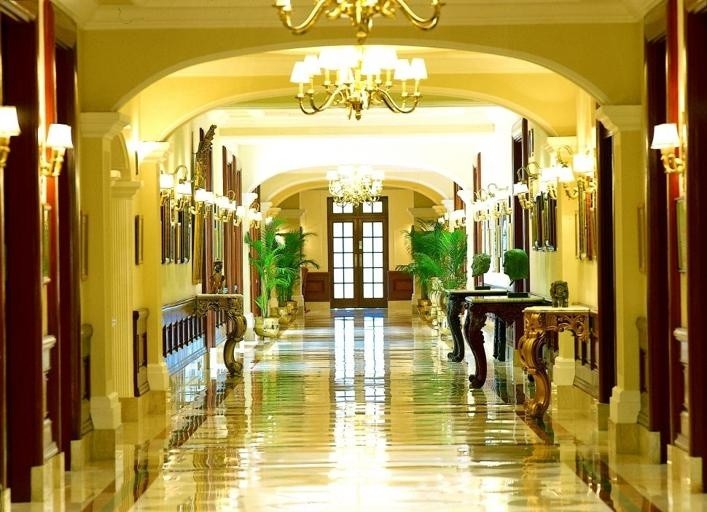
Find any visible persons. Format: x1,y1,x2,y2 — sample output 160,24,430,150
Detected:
502,248,529,280
471,252,491,277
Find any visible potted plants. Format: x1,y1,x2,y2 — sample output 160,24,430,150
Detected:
243,218,319,338
395,224,465,337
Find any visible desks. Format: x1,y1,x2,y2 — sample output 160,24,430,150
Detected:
194,293,245,374
441,287,588,419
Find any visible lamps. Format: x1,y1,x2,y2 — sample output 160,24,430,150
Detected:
1,106,74,178
273,1,446,120
649,123,683,175
